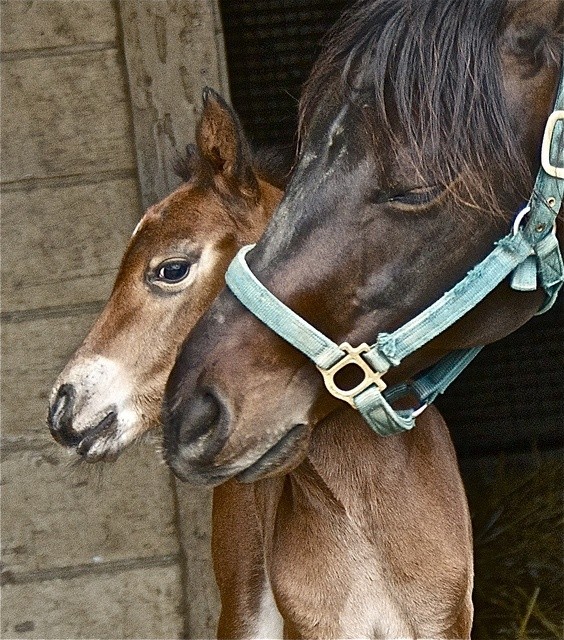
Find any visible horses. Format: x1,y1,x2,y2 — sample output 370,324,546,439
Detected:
44,85,478,639
157,0,564,493
